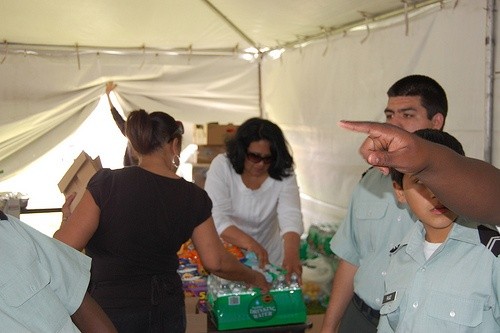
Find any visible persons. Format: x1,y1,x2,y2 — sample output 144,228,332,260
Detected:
0,210,118,333
323,74,500,333
51,82,268,333
205,116,304,286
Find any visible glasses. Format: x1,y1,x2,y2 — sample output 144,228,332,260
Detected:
166,120,185,144
243,152,274,164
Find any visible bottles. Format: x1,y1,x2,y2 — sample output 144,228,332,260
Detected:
208,264,302,298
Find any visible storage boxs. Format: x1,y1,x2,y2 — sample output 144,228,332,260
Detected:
185,121,241,190
